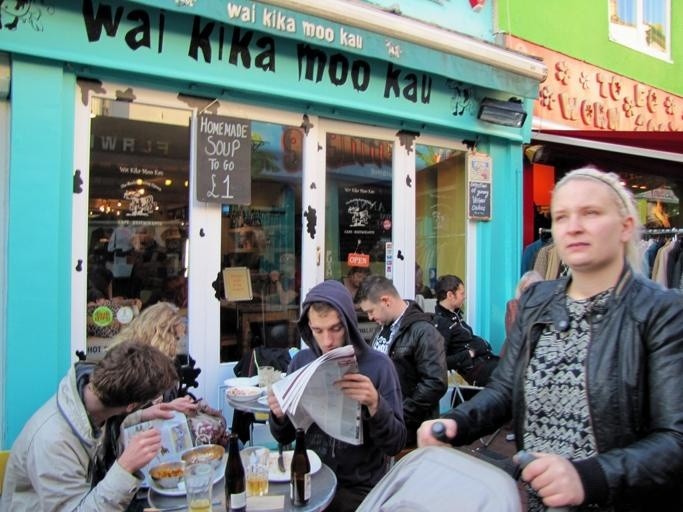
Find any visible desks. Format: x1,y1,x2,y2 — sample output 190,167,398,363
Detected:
221,300,299,361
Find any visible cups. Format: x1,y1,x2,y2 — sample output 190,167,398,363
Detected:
183,463,213,512
239,446,271,498
257,365,274,396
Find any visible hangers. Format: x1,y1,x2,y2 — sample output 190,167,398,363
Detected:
649,227,677,241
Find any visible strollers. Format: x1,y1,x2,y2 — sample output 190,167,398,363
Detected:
353,416,548,512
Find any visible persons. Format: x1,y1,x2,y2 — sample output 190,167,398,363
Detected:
1,340,197,511
269,280,406,512
500,271,543,360
416,165,682,510
354,274,448,442
432,275,498,388
88,222,372,341
108,302,185,357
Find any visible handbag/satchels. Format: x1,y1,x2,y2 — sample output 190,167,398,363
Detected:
185,408,227,444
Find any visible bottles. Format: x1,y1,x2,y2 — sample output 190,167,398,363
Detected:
290,427,311,507
227,203,284,227
223,435,247,512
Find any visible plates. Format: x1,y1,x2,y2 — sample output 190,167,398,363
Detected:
149,454,226,497
251,447,323,481
221,373,286,407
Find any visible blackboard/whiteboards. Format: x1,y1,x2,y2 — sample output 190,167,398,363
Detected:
338,179,393,262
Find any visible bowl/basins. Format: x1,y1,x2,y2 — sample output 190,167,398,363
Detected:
148,462,187,490
179,444,224,470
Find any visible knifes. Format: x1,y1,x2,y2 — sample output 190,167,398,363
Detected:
275,442,286,473
142,499,221,512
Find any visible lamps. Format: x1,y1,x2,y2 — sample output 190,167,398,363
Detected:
478,97,527,127
524,144,543,163
